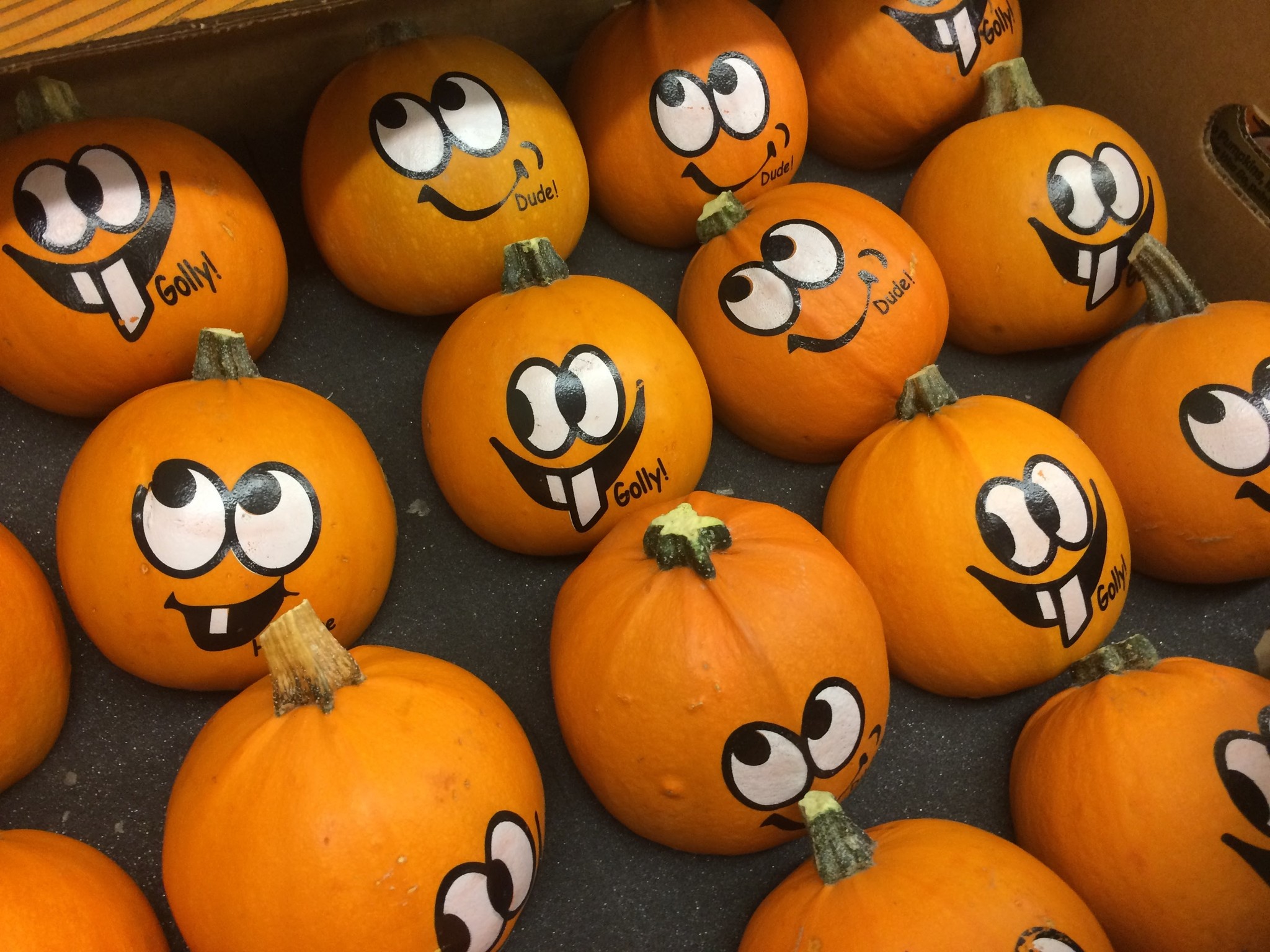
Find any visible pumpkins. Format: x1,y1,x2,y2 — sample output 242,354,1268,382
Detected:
1000,629,1267,952
786,3,1027,186
572,5,805,246
540,481,891,855
1059,222,1270,595
715,783,1097,952
147,588,537,952
50,330,404,700
891,57,1170,362
1,527,74,806
1,815,171,952
669,173,954,465
1,111,291,424
426,227,718,557
816,362,1129,703
299,35,596,311
